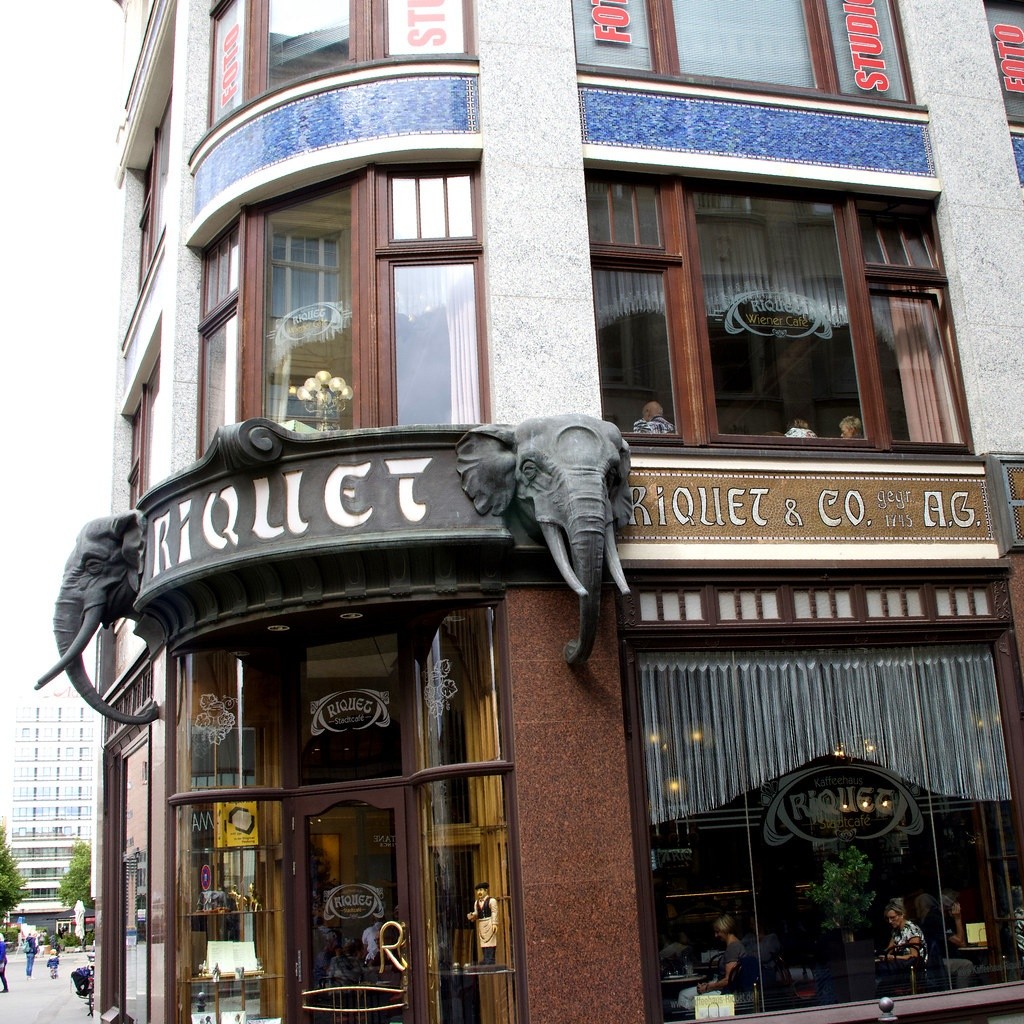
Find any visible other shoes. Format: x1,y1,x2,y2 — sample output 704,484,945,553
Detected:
0,989,8,993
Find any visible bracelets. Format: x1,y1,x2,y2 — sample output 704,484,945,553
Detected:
706,984,709,992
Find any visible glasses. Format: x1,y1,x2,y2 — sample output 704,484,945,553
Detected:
886,914,898,921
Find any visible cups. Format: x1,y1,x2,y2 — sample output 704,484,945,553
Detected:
235,967,244,979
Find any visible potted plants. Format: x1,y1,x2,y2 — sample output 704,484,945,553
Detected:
802,846,877,1002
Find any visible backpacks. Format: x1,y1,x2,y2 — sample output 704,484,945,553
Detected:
22,941,30,953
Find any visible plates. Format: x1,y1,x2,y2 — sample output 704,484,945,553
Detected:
664,976,684,978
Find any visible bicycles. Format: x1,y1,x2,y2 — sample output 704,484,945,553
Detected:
50,966,58,979
79,955,95,1017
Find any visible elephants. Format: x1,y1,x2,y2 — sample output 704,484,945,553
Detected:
34,508,159,724
454,414,631,667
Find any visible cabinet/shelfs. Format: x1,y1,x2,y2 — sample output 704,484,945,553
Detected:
180,797,286,1023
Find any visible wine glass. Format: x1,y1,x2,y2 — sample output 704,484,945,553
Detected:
256,958,264,978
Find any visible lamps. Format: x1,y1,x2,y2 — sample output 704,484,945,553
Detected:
297,370,353,431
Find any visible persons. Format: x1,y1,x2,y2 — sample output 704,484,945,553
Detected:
633,401,676,435
839,416,862,438
467,881,497,967
785,418,817,438
661,904,692,963
878,898,929,973
742,910,791,987
361,913,396,1024
47,949,59,979
314,930,367,985
1002,885,1024,982
0,933,9,993
26,931,38,980
698,914,781,1007
71,965,94,998
916,888,973,988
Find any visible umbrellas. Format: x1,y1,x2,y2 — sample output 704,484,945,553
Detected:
73,900,86,951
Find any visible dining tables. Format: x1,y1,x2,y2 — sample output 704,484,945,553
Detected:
693,965,719,971
957,947,991,988
661,974,706,1006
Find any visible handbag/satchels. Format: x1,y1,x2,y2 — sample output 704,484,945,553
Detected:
698,1002,730,1020
781,967,815,986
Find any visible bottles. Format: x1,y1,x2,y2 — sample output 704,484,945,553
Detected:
202,959,208,977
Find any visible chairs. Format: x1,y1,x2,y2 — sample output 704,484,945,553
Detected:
720,956,760,1013
770,951,809,1001
709,953,725,980
876,946,921,998
894,942,927,995
